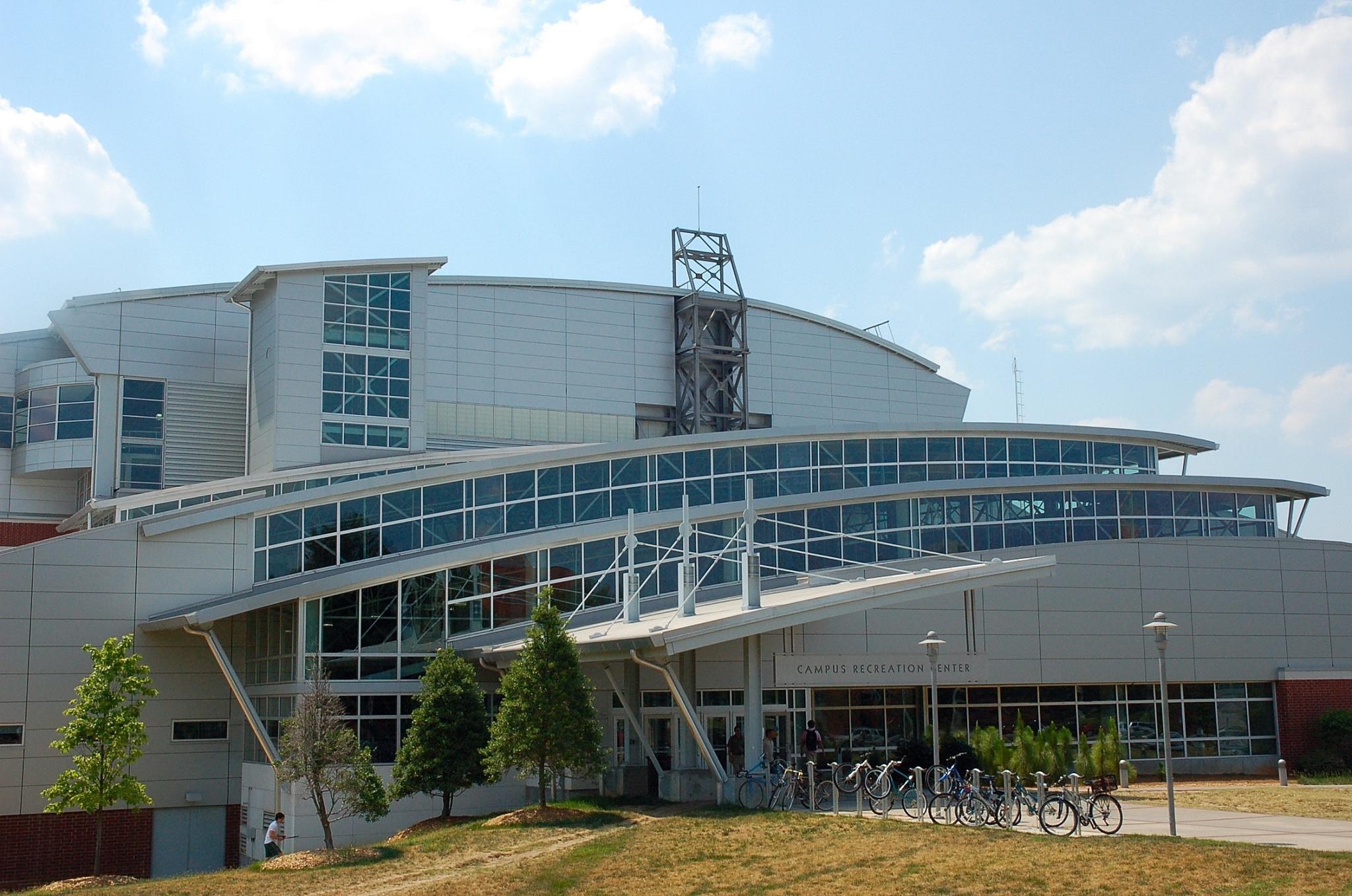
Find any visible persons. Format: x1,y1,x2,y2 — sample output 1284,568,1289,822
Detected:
264,812,286,858
727,726,744,775
800,720,827,771
762,729,778,773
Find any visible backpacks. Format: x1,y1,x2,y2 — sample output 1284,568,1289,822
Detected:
806,729,817,751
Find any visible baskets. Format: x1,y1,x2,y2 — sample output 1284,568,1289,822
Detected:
1090,774,1118,793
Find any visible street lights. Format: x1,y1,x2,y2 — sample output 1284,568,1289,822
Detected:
917,630,945,819
1141,611,1181,838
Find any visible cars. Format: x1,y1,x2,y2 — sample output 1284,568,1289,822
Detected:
834,727,915,765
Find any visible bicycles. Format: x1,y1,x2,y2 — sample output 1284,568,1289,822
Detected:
1038,773,1123,837
833,752,895,815
924,751,1069,829
736,749,839,813
864,755,928,819
